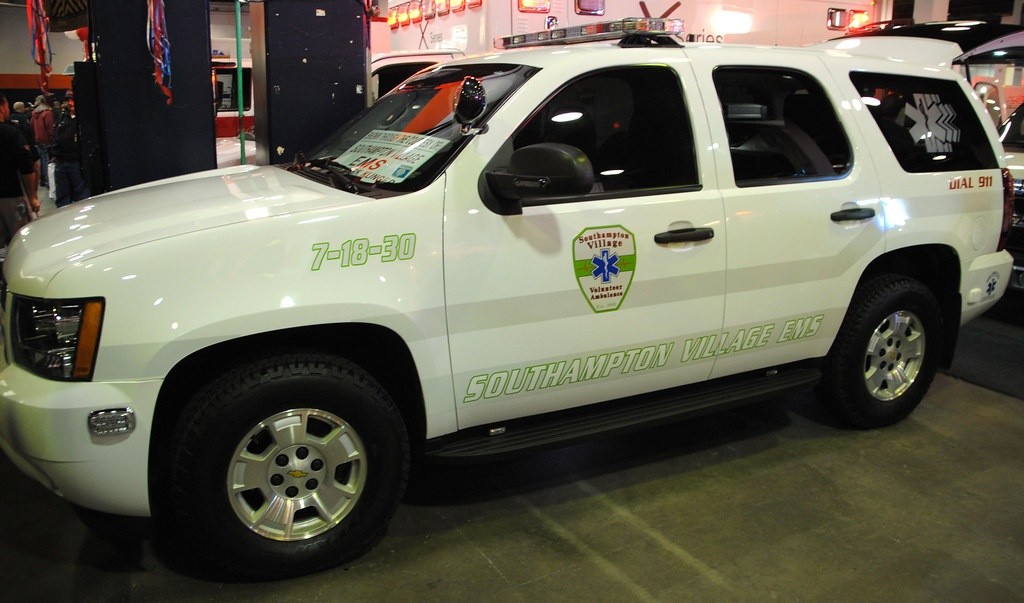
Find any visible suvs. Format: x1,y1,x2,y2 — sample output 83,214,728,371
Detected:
0,18,1016,589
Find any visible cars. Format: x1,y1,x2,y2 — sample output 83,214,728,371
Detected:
828,19,1024,306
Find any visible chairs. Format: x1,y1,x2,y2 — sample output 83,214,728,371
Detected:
591,125,686,188
544,108,596,164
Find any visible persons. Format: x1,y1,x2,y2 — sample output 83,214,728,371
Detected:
1,89,92,252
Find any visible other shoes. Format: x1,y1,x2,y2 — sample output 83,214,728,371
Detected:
40,182,50,188
